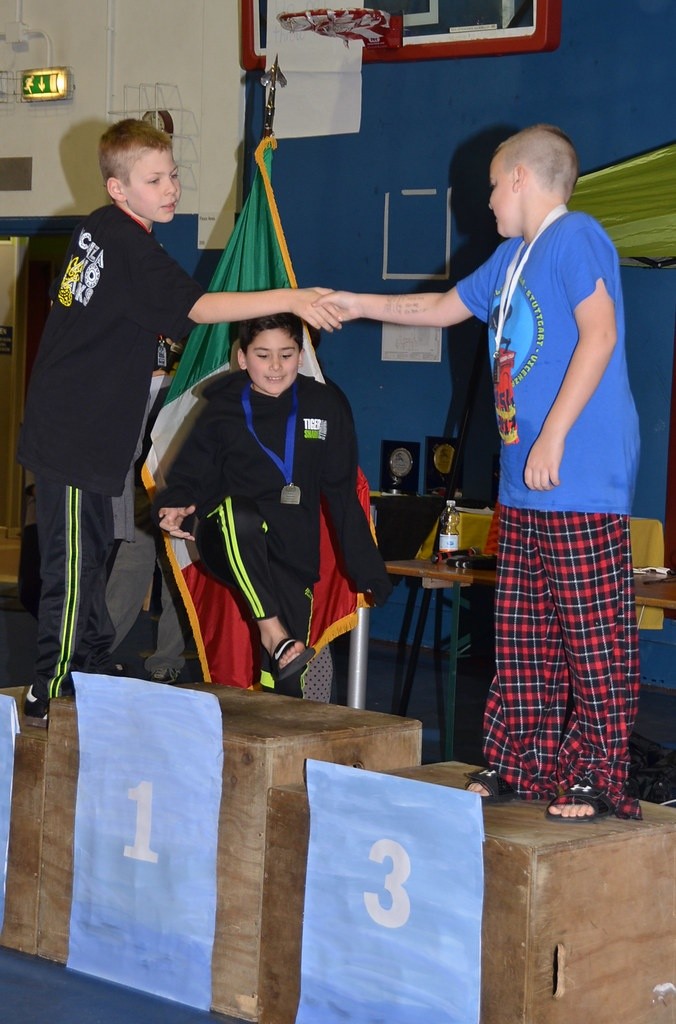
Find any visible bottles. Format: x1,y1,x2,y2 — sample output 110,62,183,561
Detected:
438,500,460,561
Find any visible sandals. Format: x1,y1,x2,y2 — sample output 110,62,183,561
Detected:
545,783,616,823
464,768,518,803
270,638,316,681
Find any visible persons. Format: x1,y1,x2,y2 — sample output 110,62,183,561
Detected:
15,118,344,728
154,312,393,699
315,122,646,822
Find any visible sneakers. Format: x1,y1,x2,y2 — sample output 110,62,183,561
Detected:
149,665,178,683
23,684,49,727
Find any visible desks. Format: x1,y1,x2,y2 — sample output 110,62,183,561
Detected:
384,558,676,765
